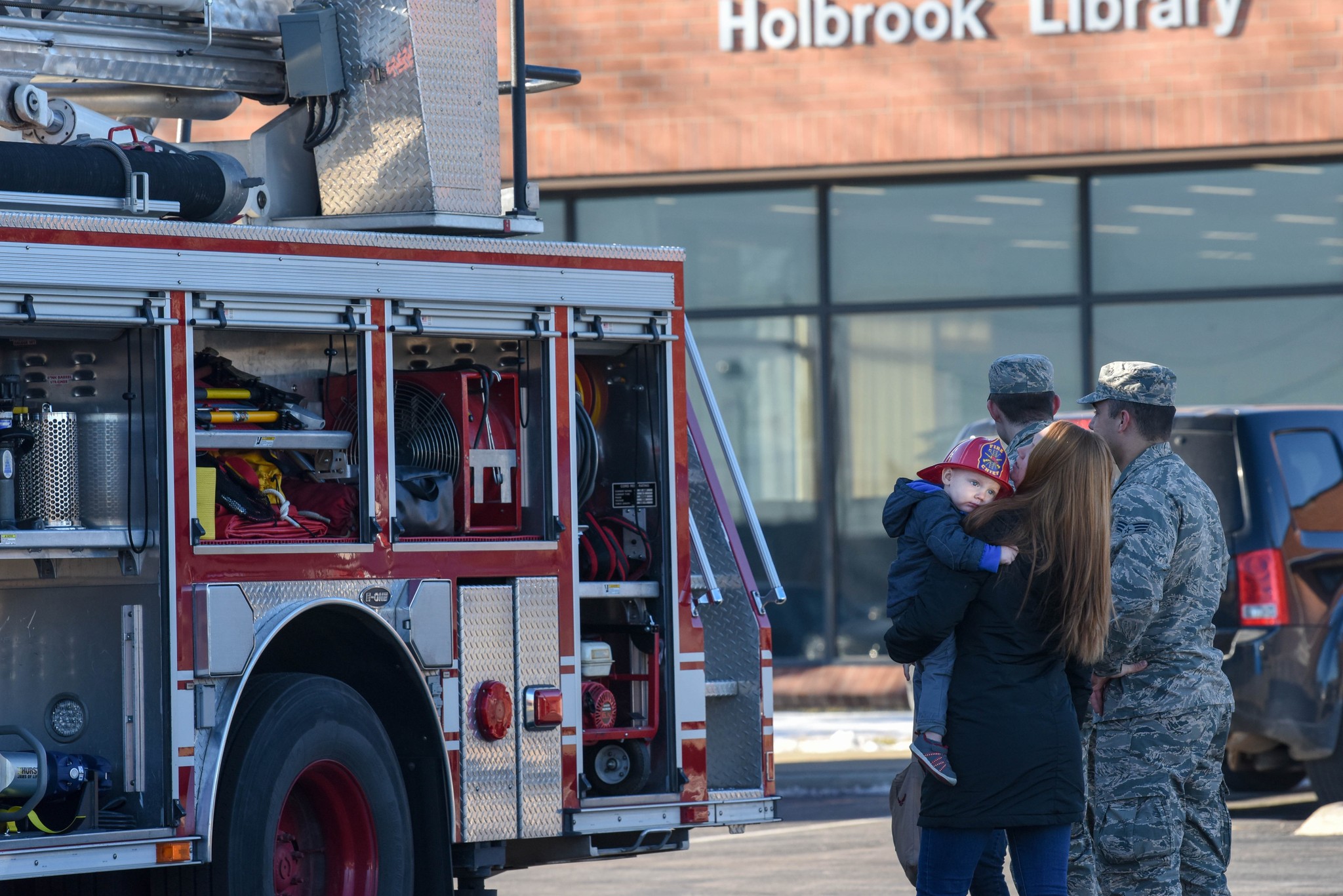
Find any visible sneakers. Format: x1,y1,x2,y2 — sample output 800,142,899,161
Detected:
910,734,958,787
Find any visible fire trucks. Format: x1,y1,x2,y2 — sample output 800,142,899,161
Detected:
0,0,787,896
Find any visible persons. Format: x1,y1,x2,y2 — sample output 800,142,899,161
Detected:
883,419,1116,896
878,438,1020,785
1079,361,1235,896
987,355,1098,896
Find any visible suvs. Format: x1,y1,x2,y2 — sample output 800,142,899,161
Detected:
955,403,1343,804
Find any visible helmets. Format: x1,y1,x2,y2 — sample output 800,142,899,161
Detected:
918,438,1012,500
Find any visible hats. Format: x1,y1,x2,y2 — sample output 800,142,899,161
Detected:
989,355,1054,394
1077,361,1176,406
